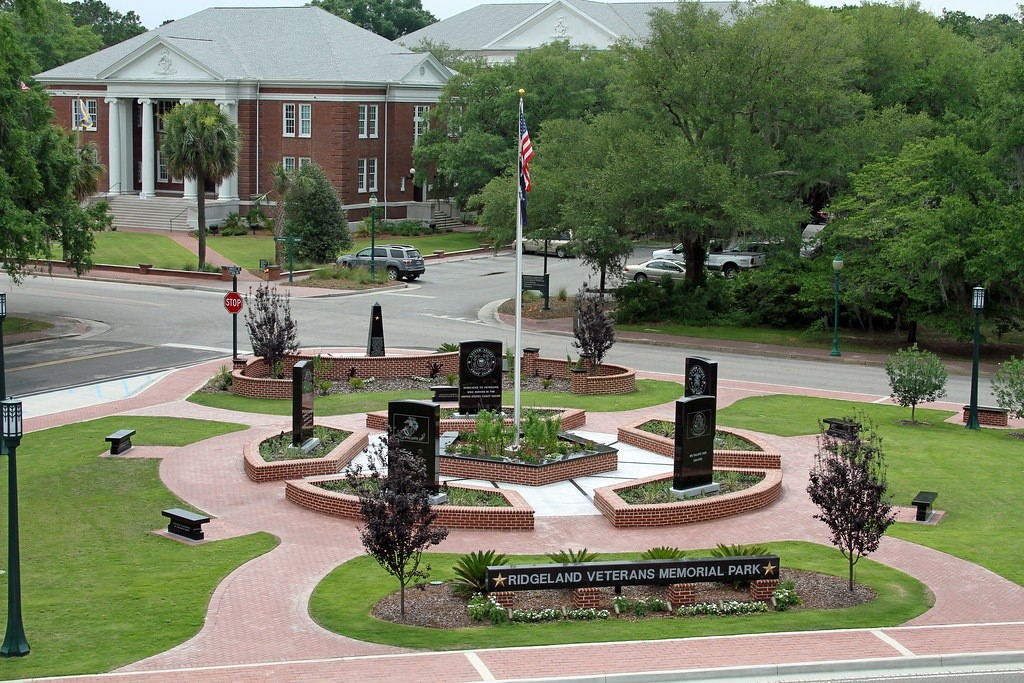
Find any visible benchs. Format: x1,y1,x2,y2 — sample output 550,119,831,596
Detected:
105,430,136,454
162,508,210,541
430,386,460,403
963,405,1010,426
911,491,939,521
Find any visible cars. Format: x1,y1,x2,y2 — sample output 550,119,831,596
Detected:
512,226,583,259
729,210,830,262
583,225,619,256
622,259,686,285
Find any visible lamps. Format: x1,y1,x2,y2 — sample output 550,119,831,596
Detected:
407,167,416,180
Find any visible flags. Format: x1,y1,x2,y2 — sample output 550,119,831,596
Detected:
520,105,536,227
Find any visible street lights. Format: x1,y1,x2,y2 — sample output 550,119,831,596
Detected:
829,253,845,356
368,193,378,283
0,396,32,658
0,292,8,401
965,284,988,430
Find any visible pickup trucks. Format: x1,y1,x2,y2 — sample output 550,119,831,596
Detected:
653,240,766,280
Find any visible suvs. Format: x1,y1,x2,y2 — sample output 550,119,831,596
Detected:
335,243,425,282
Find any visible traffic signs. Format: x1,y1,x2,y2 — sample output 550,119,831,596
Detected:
292,237,303,242
274,236,288,242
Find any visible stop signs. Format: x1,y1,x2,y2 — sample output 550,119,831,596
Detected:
223,290,244,315
